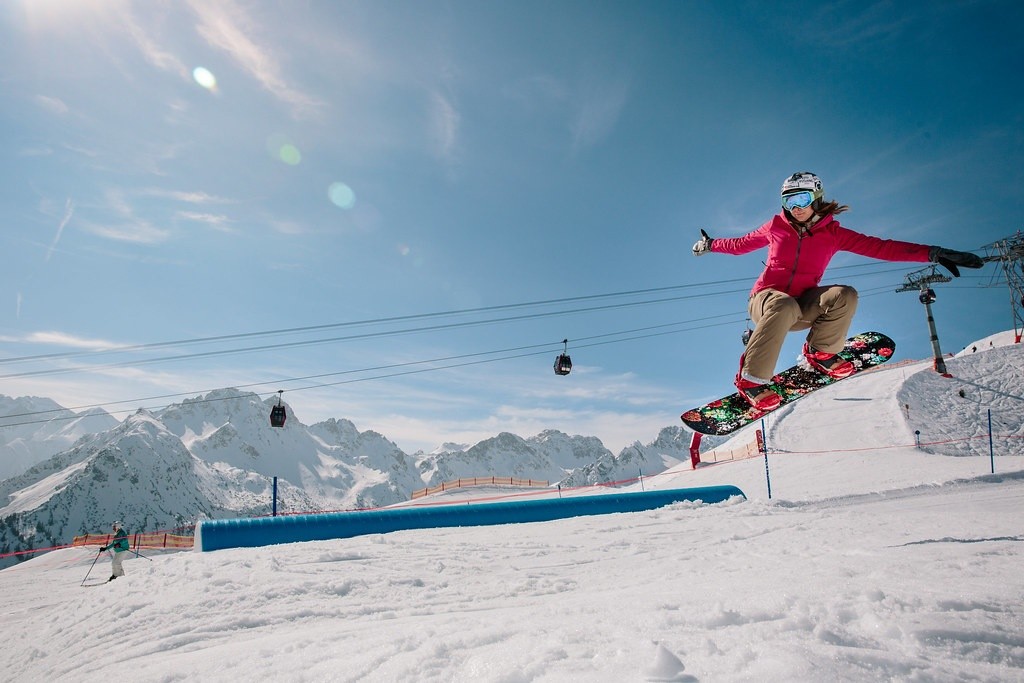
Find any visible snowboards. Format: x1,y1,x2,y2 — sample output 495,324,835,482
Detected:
680,330,896,436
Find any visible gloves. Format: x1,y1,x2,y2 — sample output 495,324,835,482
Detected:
692,228,714,256
100,547,107,551
114,543,121,548
930,246,984,278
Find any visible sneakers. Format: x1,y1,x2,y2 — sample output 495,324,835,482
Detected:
803,339,854,380
735,374,780,412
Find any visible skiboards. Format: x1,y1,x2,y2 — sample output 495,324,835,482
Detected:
81,581,109,587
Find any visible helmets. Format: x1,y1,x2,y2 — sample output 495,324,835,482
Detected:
113,521,122,531
780,171,822,196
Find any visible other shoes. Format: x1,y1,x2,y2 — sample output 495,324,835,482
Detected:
110,575,116,581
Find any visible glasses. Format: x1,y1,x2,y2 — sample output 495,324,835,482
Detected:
780,191,815,212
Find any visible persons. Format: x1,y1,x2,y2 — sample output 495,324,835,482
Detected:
100,521,129,581
972,346,977,353
693,172,984,411
